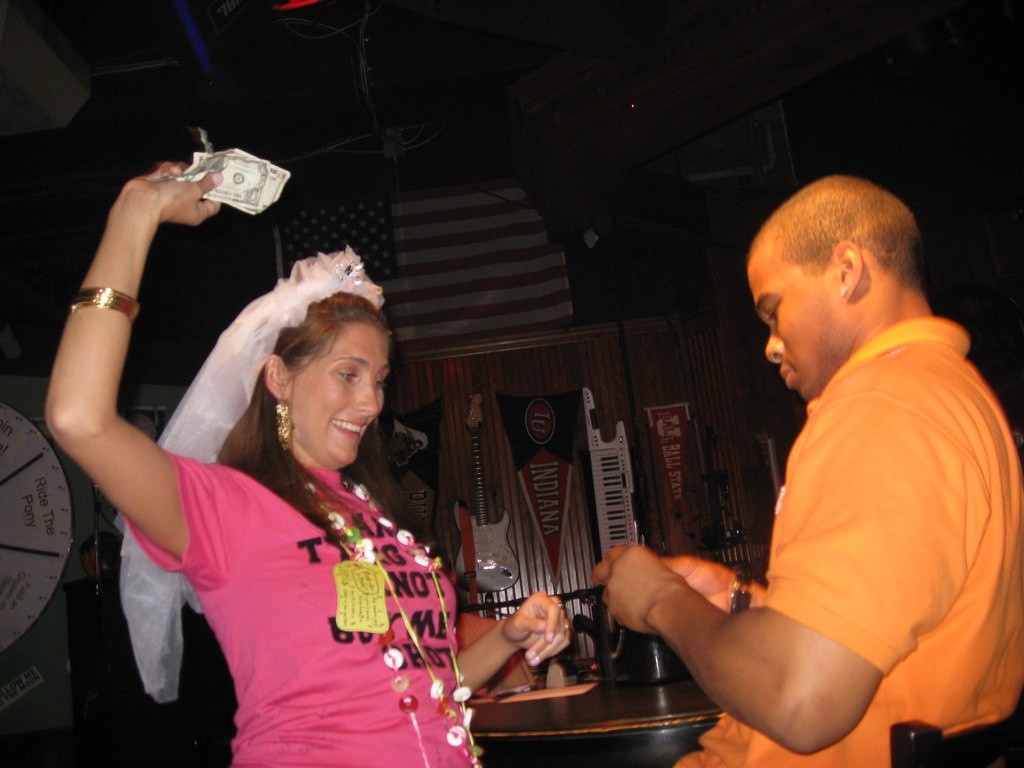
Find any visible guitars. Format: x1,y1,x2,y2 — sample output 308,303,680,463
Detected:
454,393,520,592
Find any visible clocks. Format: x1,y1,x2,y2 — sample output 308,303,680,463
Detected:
0,401,74,654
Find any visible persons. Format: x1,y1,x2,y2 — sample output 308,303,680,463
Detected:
594,174,1024,768
65,532,228,768
45,163,576,768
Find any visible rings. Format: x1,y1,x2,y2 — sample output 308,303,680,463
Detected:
557,601,565,608
560,618,570,629
571,626,575,634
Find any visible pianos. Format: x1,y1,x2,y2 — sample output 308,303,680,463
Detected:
582,385,649,566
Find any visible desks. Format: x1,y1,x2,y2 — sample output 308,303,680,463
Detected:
465,660,726,768
62,578,239,768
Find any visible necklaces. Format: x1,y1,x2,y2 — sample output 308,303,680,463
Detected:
308,478,482,768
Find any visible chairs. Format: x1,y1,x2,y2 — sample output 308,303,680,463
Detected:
891,703,1024,768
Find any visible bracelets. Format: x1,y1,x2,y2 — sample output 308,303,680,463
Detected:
71,287,140,320
730,574,753,614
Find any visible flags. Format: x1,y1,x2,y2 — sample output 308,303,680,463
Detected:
273,174,574,351
